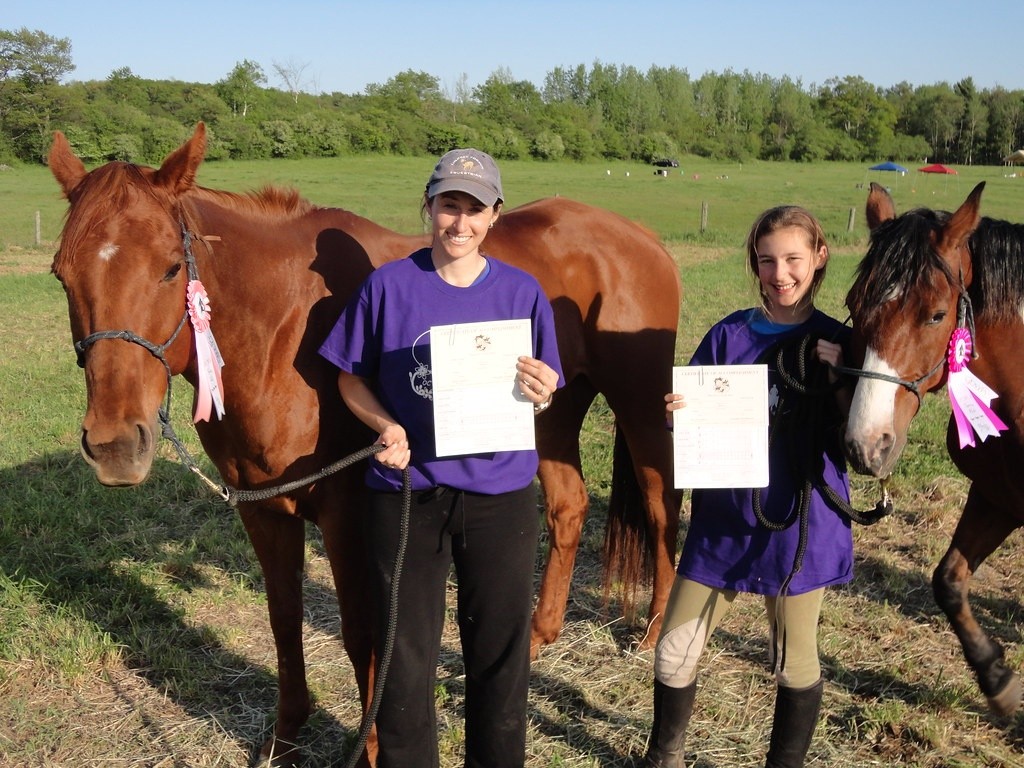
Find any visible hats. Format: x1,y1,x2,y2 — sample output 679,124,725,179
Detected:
428,148,503,207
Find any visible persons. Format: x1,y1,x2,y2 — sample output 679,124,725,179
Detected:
627,207,864,767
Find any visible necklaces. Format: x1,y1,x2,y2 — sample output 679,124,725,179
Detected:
320,149,564,768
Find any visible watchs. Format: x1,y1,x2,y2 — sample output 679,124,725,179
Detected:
534,395,550,410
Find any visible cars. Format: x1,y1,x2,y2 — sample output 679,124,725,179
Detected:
652,159,680,167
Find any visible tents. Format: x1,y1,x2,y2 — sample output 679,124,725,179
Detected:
1003,150,1024,177
861,161,909,193
913,164,958,192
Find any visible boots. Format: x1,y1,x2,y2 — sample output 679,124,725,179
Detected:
764,679,824,768
637,677,696,768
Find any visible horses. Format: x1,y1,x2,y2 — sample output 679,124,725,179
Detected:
46,123,681,767
839,180,1023,734
